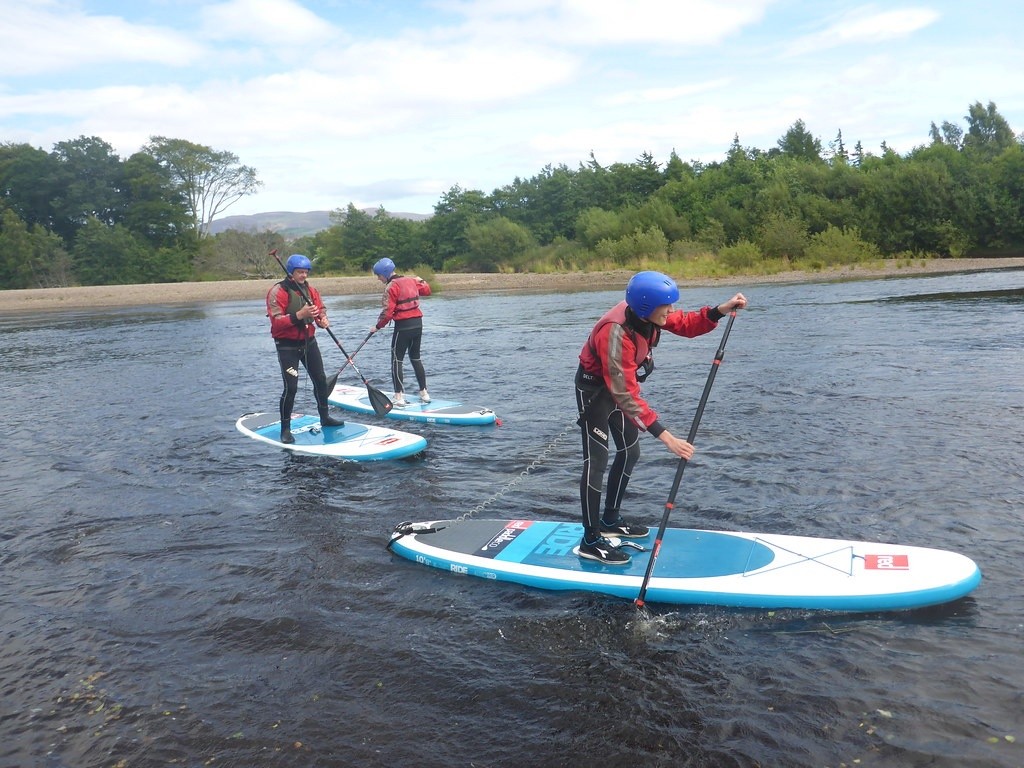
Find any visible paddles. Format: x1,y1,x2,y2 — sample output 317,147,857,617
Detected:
631,303,741,608
267,247,396,419
312,324,377,399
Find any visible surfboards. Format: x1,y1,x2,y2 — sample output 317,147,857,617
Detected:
325,383,496,428
383,515,985,612
236,409,427,468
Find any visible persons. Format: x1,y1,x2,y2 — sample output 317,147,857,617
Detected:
371,257,432,407
574,271,748,564
266,253,345,444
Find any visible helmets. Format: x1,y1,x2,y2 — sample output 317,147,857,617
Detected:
373,258,396,279
286,254,312,275
625,271,680,321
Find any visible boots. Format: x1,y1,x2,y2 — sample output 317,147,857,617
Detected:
319,407,344,426
281,418,294,443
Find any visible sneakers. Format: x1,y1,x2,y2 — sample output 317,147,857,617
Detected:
600,516,650,538
418,393,430,403
388,396,405,408
578,535,632,565
